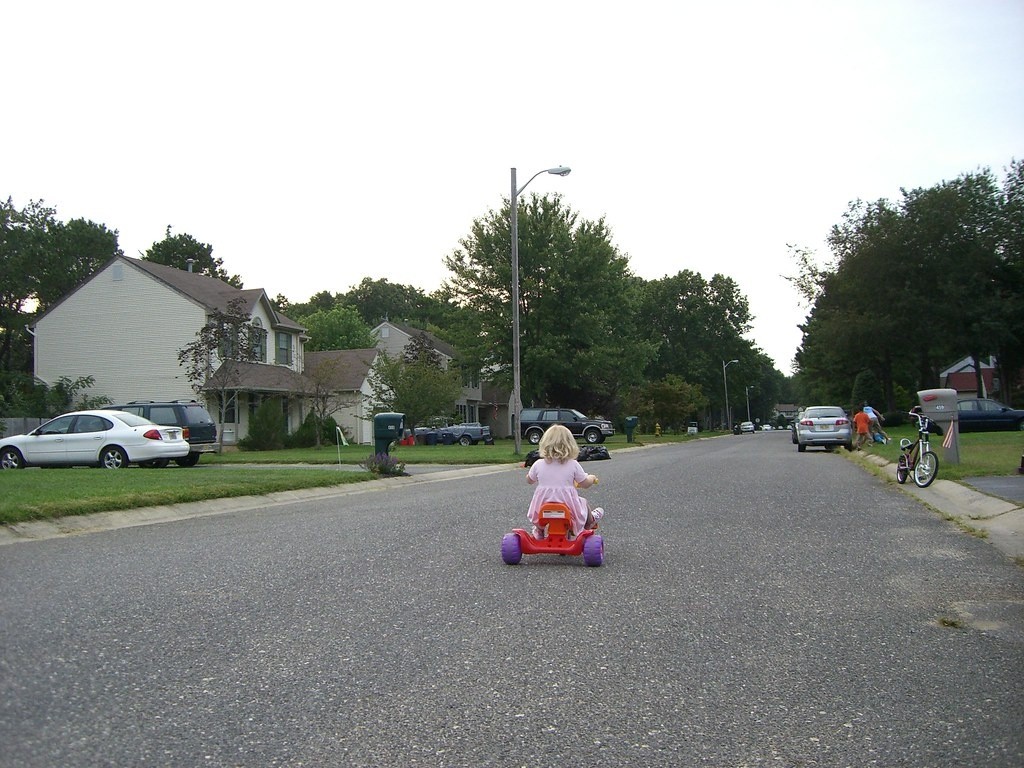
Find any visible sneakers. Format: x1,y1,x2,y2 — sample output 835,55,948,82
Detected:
584,507,604,530
532,525,544,540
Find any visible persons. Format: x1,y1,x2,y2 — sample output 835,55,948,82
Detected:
525,425,605,540
863,400,891,444
854,406,874,451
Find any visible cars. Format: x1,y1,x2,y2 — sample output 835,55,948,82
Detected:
957,398,1024,433
790,406,852,452
760,424,772,431
0,410,190,469
739,421,755,434
520,407,546,420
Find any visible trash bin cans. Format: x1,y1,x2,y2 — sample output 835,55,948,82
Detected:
442,432,453,445
688,422,698,436
427,432,438,446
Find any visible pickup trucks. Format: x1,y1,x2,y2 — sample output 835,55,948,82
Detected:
520,409,616,445
404,416,490,446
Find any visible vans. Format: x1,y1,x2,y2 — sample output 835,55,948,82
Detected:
100,399,220,468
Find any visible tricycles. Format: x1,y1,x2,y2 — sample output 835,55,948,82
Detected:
501,502,604,567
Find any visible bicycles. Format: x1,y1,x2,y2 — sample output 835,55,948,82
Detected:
897,409,939,488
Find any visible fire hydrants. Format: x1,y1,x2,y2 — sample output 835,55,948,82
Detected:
654,423,661,438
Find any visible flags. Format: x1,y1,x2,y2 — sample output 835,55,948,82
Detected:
943,421,953,447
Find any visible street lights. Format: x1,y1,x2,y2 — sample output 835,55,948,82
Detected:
722,360,738,431
745,386,754,422
510,167,571,454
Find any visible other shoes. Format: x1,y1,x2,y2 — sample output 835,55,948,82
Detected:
886,437,891,444
858,447,861,451
866,443,873,447
883,439,886,445
